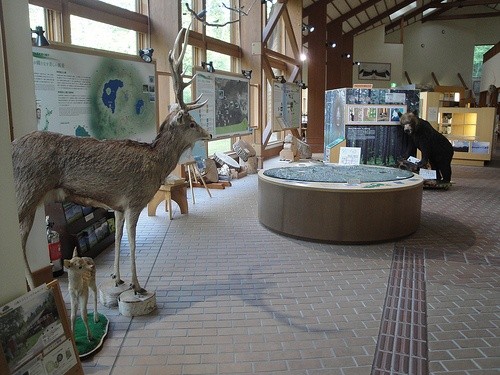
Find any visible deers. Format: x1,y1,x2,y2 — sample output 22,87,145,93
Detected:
11,20,213,343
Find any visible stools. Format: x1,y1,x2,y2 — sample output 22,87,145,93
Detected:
148,181,190,220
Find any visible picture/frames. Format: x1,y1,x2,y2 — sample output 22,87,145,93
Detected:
358,62,391,81
345,104,407,125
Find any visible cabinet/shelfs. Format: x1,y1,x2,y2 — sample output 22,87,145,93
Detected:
419,91,445,131
437,106,496,167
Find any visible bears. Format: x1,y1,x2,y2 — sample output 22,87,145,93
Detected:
396,109,454,184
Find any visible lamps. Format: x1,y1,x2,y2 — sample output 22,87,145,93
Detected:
276,75,286,84
30,26,51,47
341,51,352,59
294,80,305,88
201,60,215,73
242,70,252,79
326,41,337,49
353,61,361,67
261,0,278,5
303,22,315,33
139,48,155,63
